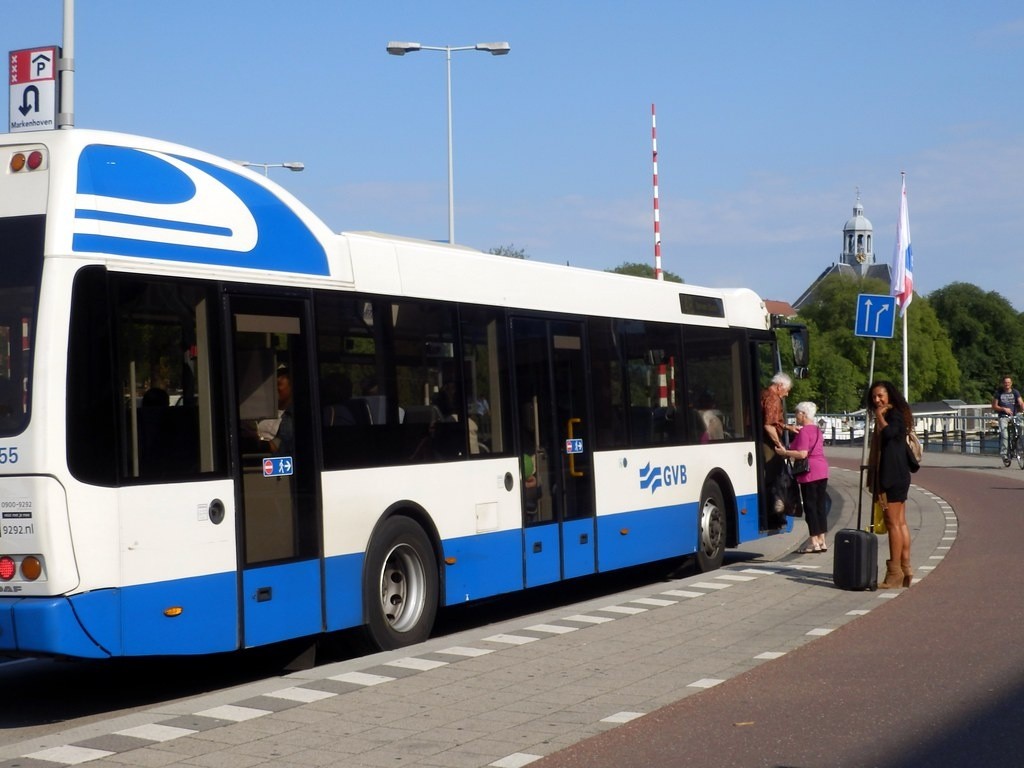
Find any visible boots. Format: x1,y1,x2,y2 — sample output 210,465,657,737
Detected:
876,560,904,590
901,559,913,588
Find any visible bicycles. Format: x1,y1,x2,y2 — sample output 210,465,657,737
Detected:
999,413,1024,471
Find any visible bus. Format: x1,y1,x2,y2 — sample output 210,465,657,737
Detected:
2,129,803,661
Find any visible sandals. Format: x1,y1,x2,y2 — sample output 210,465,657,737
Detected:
819,544,827,552
798,545,821,553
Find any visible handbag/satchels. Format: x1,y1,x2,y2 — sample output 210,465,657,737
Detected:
873,500,888,534
772,458,800,504
905,425,922,473
791,457,809,475
785,497,802,517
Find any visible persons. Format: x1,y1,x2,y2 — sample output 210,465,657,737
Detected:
140,363,458,469
866,380,914,587
761,373,829,554
525,476,539,518
994,376,1024,463
476,393,490,442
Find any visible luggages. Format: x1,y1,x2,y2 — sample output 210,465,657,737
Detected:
832,465,879,592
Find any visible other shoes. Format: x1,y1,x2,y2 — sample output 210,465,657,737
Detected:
1002,455,1008,461
768,516,787,524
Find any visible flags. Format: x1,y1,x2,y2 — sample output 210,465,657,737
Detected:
890,186,913,317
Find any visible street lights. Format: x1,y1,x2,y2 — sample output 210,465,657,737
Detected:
385,41,515,244
230,159,306,181
